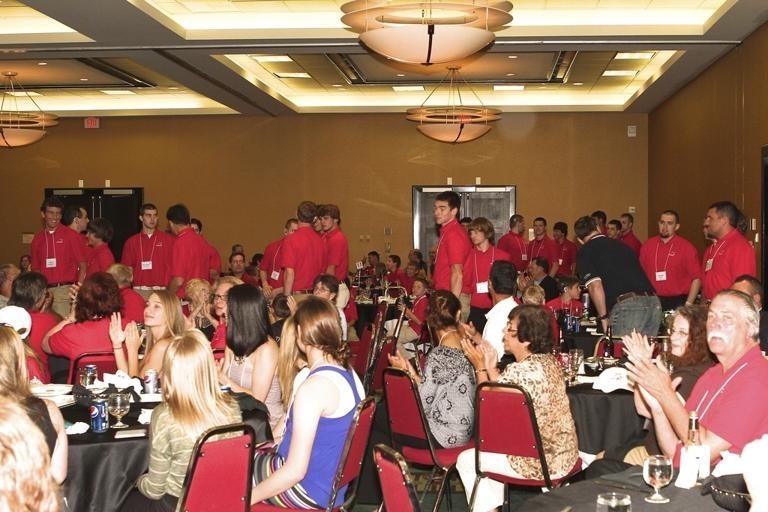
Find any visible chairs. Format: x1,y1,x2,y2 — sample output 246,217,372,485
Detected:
467,381,552,510
254,396,377,512
174,422,258,511
369,442,428,511
4,266,768,385
378,366,476,512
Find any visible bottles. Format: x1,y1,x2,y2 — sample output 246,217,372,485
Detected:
684,411,703,484
603,325,614,356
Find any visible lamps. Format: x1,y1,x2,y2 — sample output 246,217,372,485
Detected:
0,70,59,149
340,0,514,66
406,65,502,143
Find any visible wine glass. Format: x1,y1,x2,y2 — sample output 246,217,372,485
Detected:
570,349,584,382
109,393,130,428
643,454,673,503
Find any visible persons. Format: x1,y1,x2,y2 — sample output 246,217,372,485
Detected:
365,250,768,488
260,200,350,322
577,201,759,337
120,204,221,315
29,197,116,317
433,191,576,335
0,244,366,511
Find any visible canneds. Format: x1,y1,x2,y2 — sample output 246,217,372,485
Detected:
136,323,143,334
375,278,381,286
372,294,379,305
563,313,581,333
89,398,110,434
83,365,98,386
143,369,159,396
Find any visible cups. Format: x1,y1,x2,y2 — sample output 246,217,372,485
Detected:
647,334,671,362
365,268,369,275
595,493,633,511
79,369,97,389
558,289,595,336
373,294,378,304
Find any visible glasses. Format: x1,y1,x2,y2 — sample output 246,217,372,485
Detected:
313,286,329,292
507,321,518,332
213,294,228,301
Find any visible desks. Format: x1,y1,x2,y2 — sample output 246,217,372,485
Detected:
522,460,768,512
556,353,662,456
22,382,269,512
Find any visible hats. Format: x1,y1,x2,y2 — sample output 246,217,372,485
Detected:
1,306,32,340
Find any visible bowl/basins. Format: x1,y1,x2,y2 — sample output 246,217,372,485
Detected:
710,472,751,512
586,354,617,375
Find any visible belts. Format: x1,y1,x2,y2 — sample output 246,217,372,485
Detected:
291,288,312,295
612,292,657,305
46,282,73,288
